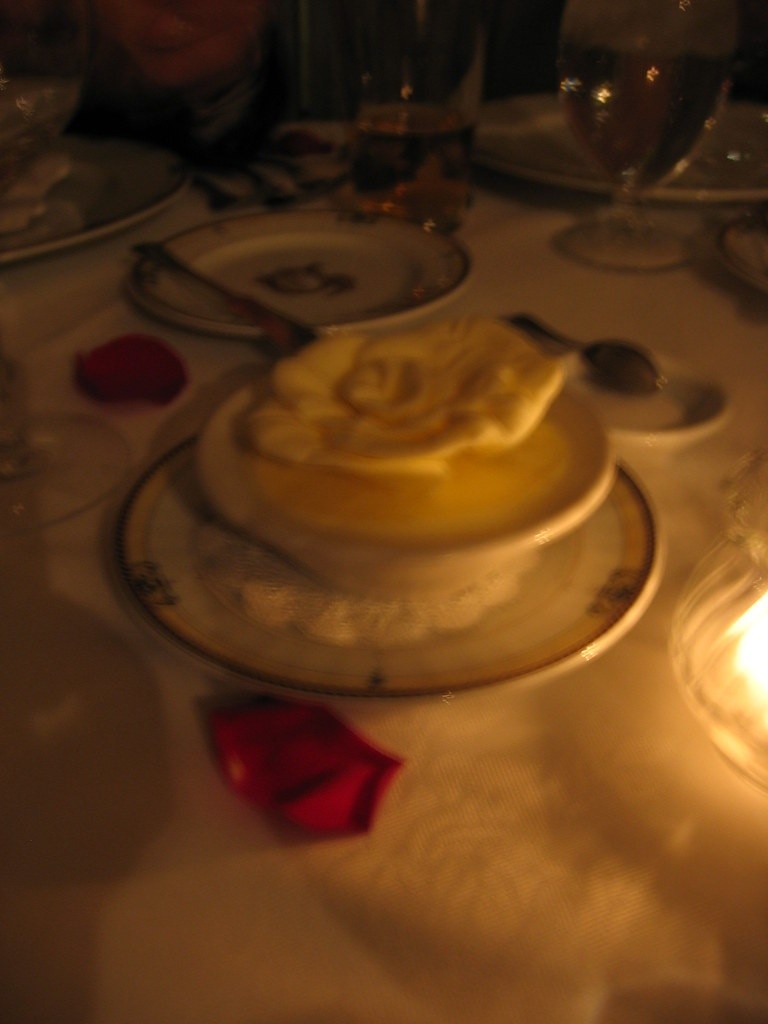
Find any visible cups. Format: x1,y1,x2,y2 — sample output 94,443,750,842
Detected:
351,0,482,232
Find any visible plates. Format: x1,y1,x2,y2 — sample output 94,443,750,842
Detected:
0,140,189,263
555,350,732,442
127,210,471,336
113,432,665,705
474,105,768,198
716,217,768,289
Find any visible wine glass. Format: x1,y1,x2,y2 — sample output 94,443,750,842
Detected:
551,0,739,270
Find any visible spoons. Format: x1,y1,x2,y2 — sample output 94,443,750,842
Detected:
511,315,662,391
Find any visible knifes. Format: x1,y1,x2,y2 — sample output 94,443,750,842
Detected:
133,242,313,345
